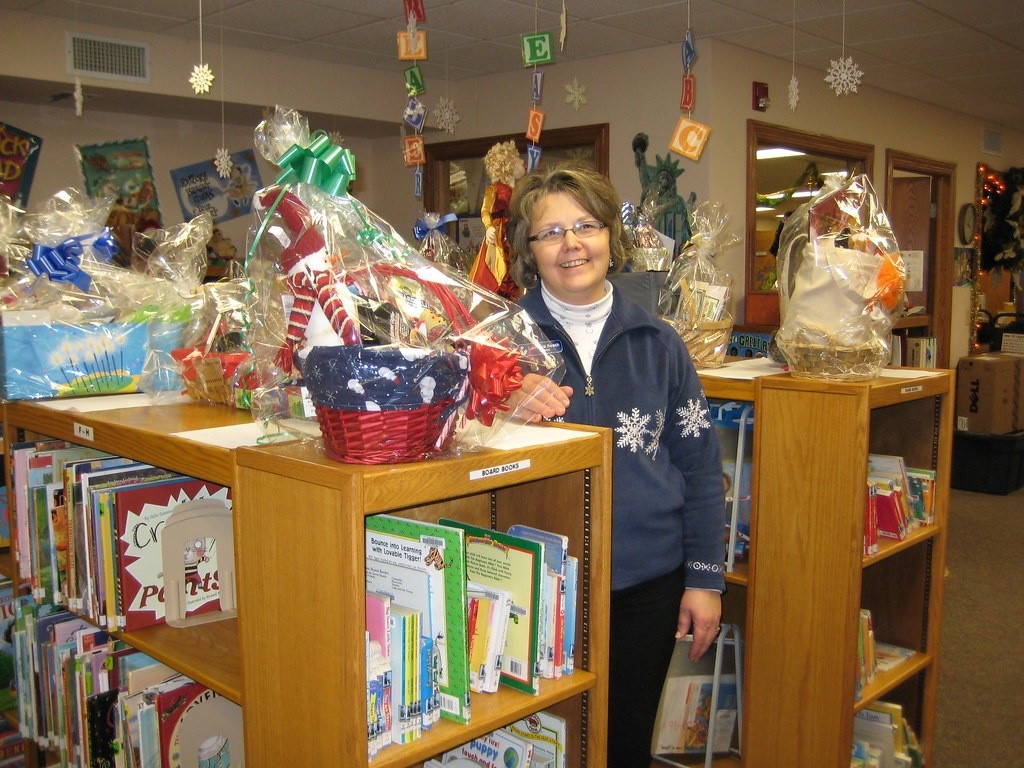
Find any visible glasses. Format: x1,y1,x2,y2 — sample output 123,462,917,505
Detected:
528,221,610,246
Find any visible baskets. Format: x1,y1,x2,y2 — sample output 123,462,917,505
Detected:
658,279,735,369
292,264,481,467
774,322,895,381
177,351,253,411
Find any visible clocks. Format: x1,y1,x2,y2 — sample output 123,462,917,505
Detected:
959,203,975,245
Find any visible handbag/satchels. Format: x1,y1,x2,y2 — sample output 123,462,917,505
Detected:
780,229,894,347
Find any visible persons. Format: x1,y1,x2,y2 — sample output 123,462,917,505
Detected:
458,156,727,768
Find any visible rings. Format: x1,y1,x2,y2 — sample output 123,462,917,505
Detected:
712,625,721,633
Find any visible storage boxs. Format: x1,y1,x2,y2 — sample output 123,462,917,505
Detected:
952,431,1024,496
957,351,1024,434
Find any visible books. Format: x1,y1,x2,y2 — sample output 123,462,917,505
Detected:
0,420,583,767
891,333,938,370
648,395,939,767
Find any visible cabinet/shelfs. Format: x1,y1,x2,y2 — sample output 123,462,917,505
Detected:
0,391,613,768
651,356,956,768
